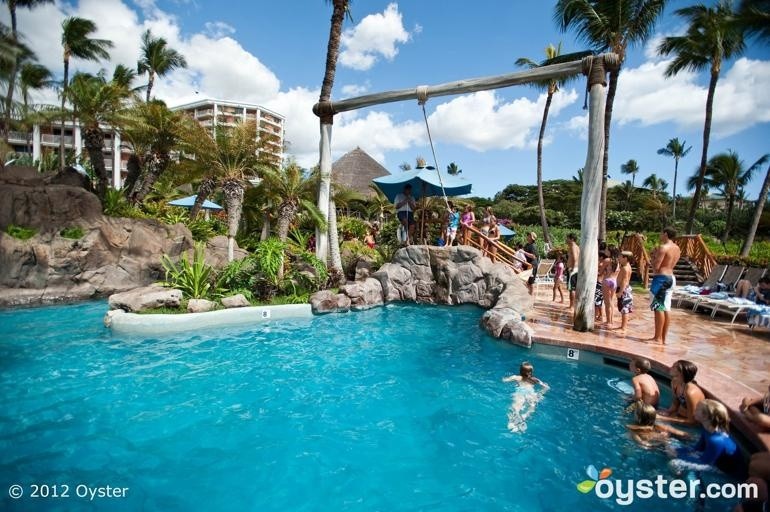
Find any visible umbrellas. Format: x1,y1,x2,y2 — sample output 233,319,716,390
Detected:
166,194,224,210
370,163,473,245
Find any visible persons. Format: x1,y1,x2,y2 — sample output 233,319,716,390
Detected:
502,361,550,435
305,186,682,347
734,275,770,307
624,357,770,511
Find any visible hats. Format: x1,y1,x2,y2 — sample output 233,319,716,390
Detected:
531,232,537,240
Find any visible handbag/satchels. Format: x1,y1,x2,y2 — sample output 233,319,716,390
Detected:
429,240,445,246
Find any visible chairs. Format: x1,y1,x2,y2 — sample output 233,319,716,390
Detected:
532,257,555,289
673,261,770,332
